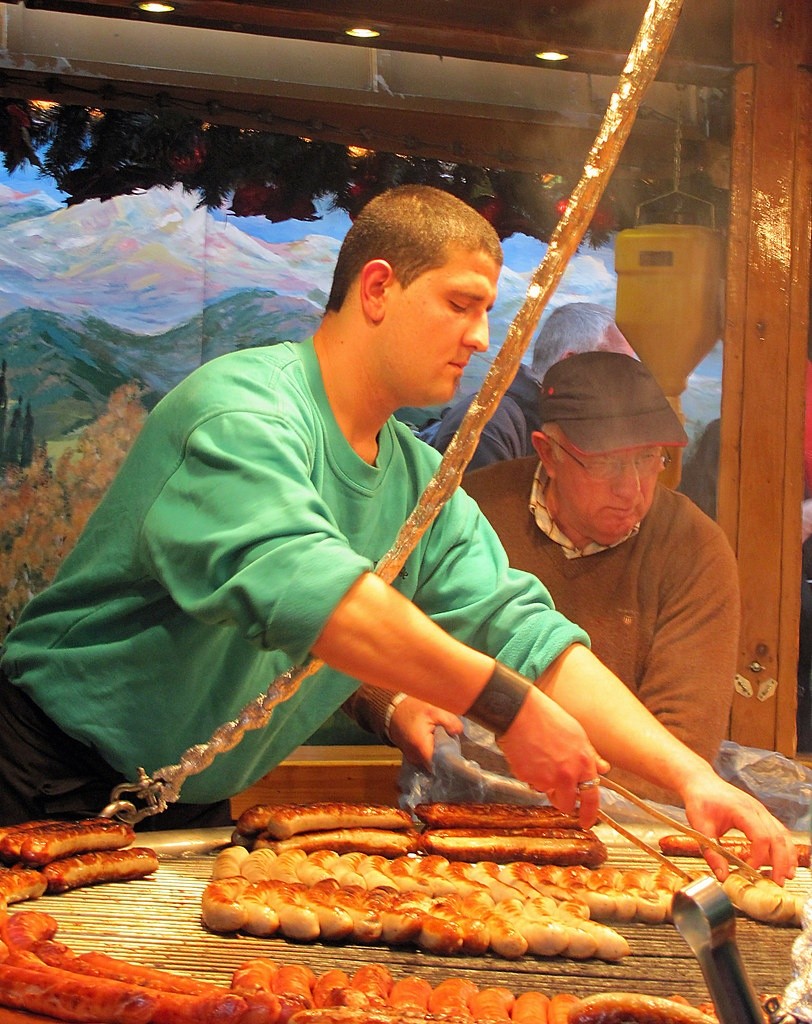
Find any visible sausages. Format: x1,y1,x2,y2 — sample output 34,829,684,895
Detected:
0,801,812,1024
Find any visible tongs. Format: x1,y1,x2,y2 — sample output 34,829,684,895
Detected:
571,774,780,912
671,877,766,1024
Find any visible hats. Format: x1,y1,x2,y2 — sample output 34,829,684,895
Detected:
539,351,690,455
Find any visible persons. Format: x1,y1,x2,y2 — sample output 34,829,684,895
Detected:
0,182,812,885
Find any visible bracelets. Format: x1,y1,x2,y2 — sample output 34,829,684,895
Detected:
462,659,534,738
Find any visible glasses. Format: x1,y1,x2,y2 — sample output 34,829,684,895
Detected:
535,429,671,482
